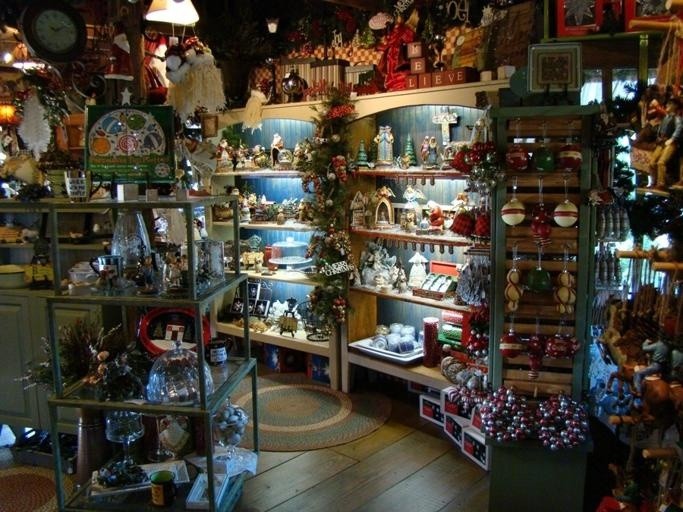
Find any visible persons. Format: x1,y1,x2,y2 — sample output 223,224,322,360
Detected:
215,124,304,168
602,83,683,424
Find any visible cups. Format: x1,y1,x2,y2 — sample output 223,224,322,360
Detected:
150,470,179,507
89,255,124,278
205,338,235,366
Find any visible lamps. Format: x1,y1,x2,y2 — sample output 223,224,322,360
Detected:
144,0,200,27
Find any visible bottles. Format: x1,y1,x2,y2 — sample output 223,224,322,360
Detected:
423,316,439,368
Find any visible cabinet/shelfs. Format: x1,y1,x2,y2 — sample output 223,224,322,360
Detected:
464,98,599,498
585,78,683,468
47,194,261,512
1,190,115,438
198,78,513,399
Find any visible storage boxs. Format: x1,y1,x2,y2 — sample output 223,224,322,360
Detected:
403,380,489,474
